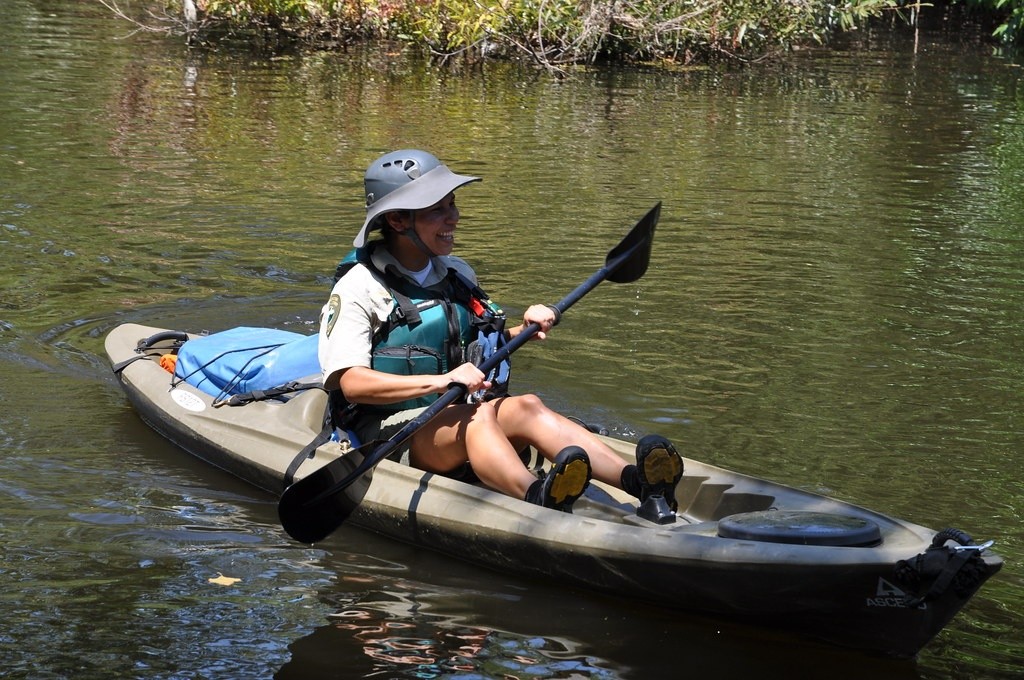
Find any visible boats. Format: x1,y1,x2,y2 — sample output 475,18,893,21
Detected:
105,320,1004,663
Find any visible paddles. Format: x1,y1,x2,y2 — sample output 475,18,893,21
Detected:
279,202,664,544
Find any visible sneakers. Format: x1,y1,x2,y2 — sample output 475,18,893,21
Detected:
621,435,683,512
526,446,592,514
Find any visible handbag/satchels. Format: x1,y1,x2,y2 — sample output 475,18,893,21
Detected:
465,309,513,404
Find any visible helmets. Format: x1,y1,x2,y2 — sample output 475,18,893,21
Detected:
352,147,485,248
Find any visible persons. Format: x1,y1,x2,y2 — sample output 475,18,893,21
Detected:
318,149,684,520
